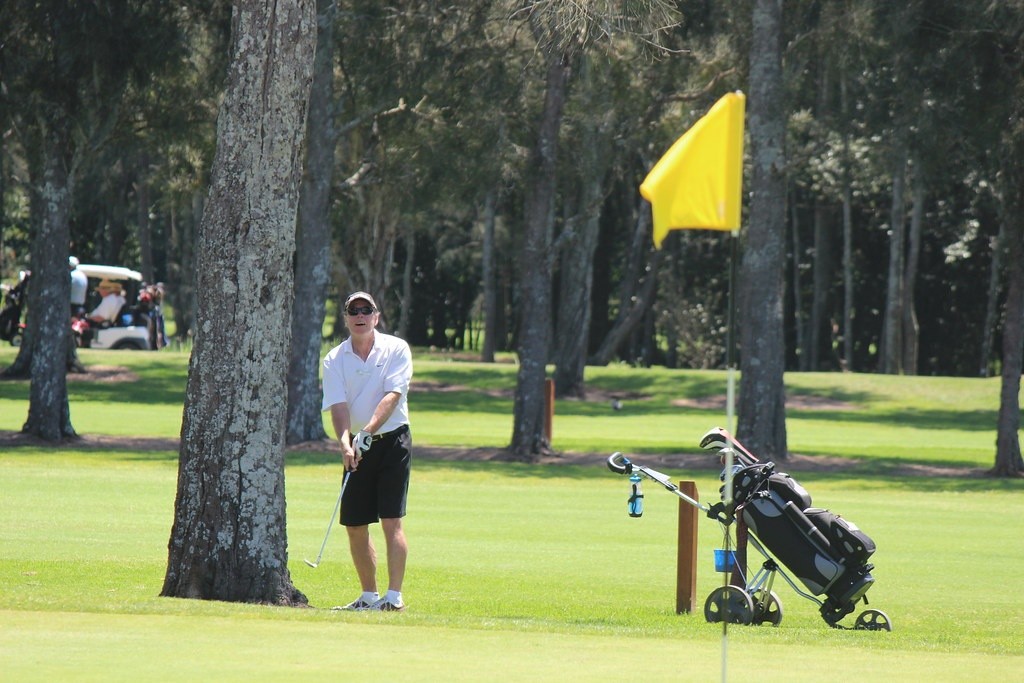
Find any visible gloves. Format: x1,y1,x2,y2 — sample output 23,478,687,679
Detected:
351,430,373,461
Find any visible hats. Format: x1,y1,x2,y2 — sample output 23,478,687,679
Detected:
94,280,112,291
113,283,126,295
69,256,79,267
345,291,377,313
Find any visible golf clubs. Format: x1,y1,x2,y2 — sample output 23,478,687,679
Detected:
716,448,748,503
301,429,372,569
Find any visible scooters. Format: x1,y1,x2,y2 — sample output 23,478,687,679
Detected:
0,289,93,348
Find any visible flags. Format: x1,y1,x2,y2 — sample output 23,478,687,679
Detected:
640,91,744,248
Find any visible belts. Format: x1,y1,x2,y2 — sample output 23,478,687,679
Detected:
349,425,407,441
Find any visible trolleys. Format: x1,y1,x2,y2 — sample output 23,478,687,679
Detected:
607,429,891,630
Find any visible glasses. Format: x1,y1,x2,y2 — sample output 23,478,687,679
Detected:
347,306,374,316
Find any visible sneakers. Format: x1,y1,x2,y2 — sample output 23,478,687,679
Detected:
330,597,370,610
355,596,405,612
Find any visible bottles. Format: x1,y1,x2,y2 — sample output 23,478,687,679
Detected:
628,474,643,517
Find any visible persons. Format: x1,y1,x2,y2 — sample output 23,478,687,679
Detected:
70,256,168,346
322,291,413,612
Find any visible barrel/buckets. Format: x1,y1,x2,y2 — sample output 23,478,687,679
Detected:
714,549,737,572
